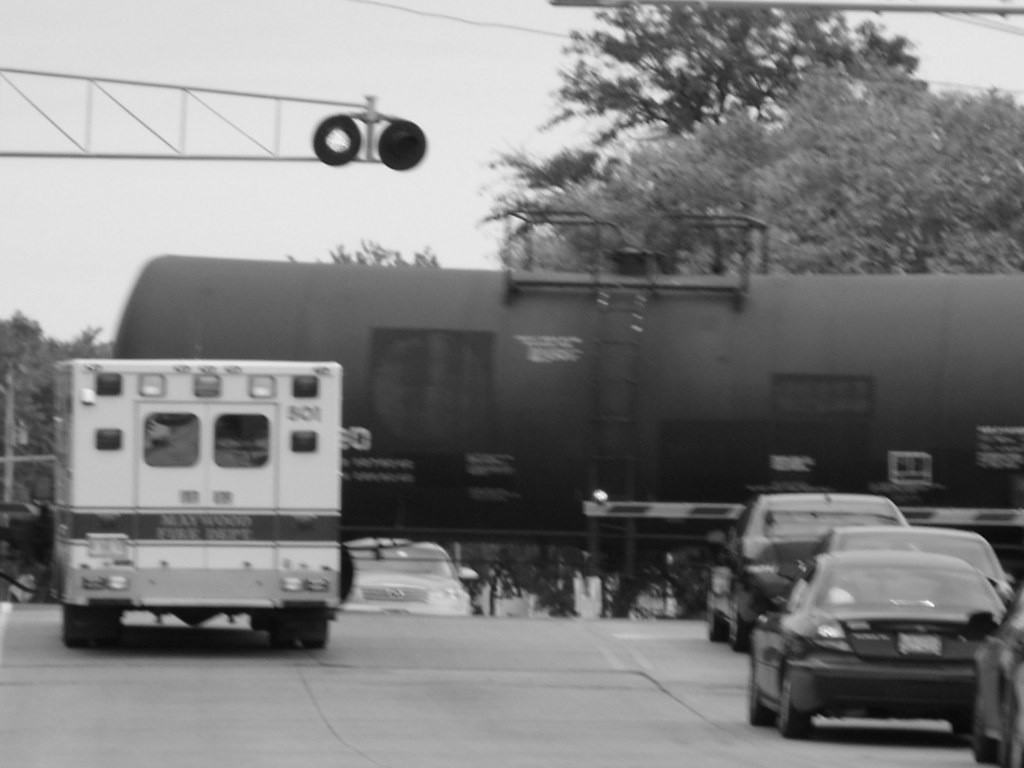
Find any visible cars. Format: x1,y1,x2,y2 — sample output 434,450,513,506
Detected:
963,576,1024,768
744,546,1017,741
0,496,52,556
777,524,1021,615
332,535,485,619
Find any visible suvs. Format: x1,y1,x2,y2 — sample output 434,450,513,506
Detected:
700,492,914,663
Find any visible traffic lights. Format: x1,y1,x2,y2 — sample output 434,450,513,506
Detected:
379,118,427,169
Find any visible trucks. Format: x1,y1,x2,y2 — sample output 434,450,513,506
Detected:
49,350,374,654
